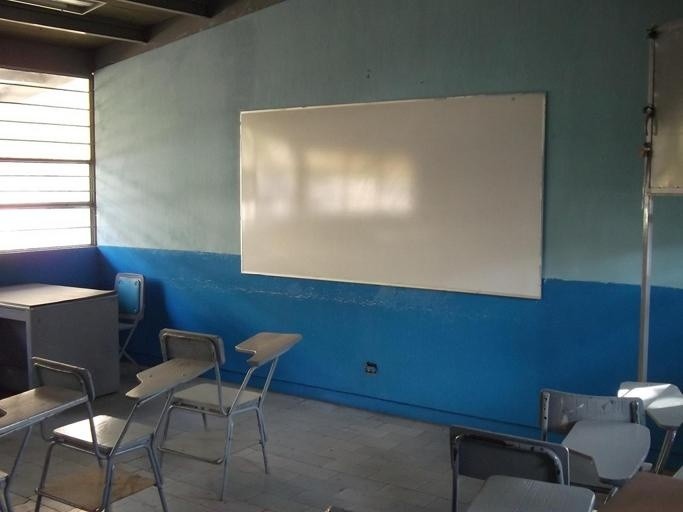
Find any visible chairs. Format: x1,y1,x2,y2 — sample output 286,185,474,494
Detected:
157,327,301,502
112,273,147,371
448,380,682,511
27,354,218,511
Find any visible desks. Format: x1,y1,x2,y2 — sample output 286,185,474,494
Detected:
0,284,120,398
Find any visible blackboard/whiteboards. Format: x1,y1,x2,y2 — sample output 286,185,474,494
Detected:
645,24,683,195
240,92,546,299
